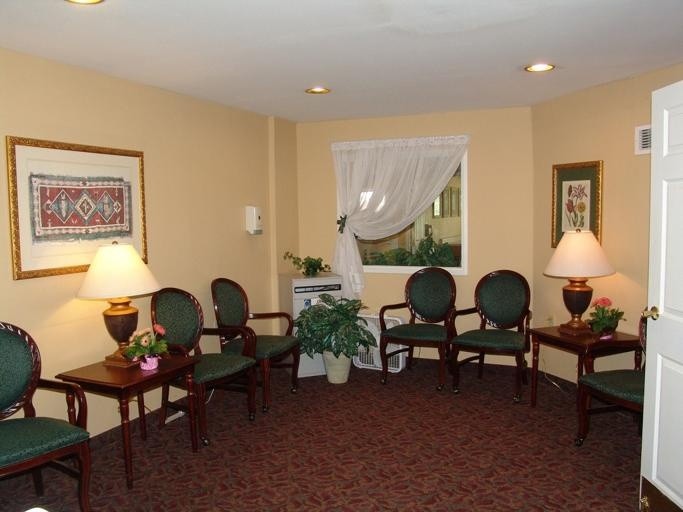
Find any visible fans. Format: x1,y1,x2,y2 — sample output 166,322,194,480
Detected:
351,311,405,374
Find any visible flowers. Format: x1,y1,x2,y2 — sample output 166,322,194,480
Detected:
586,296,627,338
120,324,168,359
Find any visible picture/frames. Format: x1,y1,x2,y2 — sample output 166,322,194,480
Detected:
5,134,148,281
432,185,464,220
550,160,604,250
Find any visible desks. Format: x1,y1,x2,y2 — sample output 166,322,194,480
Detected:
53,350,201,491
528,322,643,423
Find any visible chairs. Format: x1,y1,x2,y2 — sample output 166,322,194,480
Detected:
210,276,301,414
450,268,533,403
151,287,260,453
0,320,95,512
574,306,647,448
381,267,456,395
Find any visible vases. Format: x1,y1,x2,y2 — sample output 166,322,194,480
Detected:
141,358,158,367
588,323,618,335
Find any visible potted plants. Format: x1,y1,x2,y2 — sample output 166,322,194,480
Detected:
282,249,332,276
292,292,379,387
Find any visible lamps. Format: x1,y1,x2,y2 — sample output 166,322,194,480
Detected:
542,229,616,337
75,241,162,369
